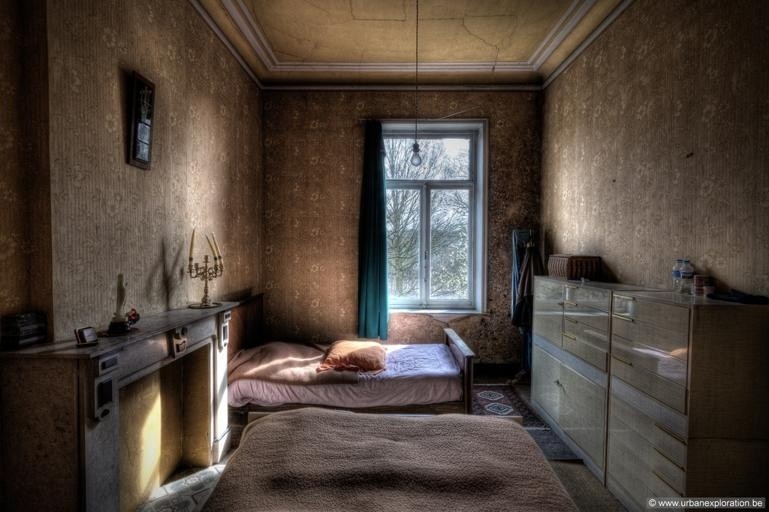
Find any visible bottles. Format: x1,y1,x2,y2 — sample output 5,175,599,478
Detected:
672,259,696,296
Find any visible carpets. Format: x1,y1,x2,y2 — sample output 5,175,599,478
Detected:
471,383,552,430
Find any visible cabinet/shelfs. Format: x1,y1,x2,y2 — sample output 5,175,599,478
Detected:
529,274,769,512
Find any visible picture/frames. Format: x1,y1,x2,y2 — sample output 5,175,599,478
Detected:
128,69,156,171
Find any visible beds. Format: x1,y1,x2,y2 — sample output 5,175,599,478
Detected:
224,292,476,447
201,407,579,512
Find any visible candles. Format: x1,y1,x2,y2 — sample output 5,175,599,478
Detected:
189,228,195,256
205,232,220,257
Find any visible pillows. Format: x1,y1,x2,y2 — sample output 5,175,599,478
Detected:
316,338,388,376
